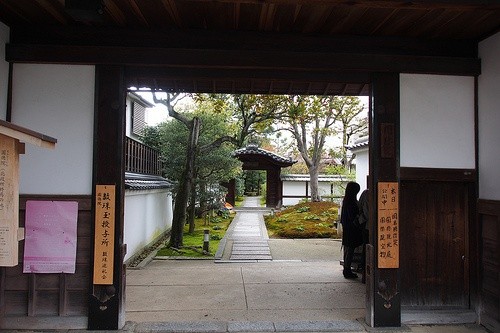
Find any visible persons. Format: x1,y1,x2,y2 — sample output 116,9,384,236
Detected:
357,188,370,283
340,182,361,278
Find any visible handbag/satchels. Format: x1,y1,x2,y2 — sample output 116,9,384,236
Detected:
353,215,368,230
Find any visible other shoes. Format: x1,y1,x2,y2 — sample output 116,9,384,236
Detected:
341,271,358,279
340,260,345,266
